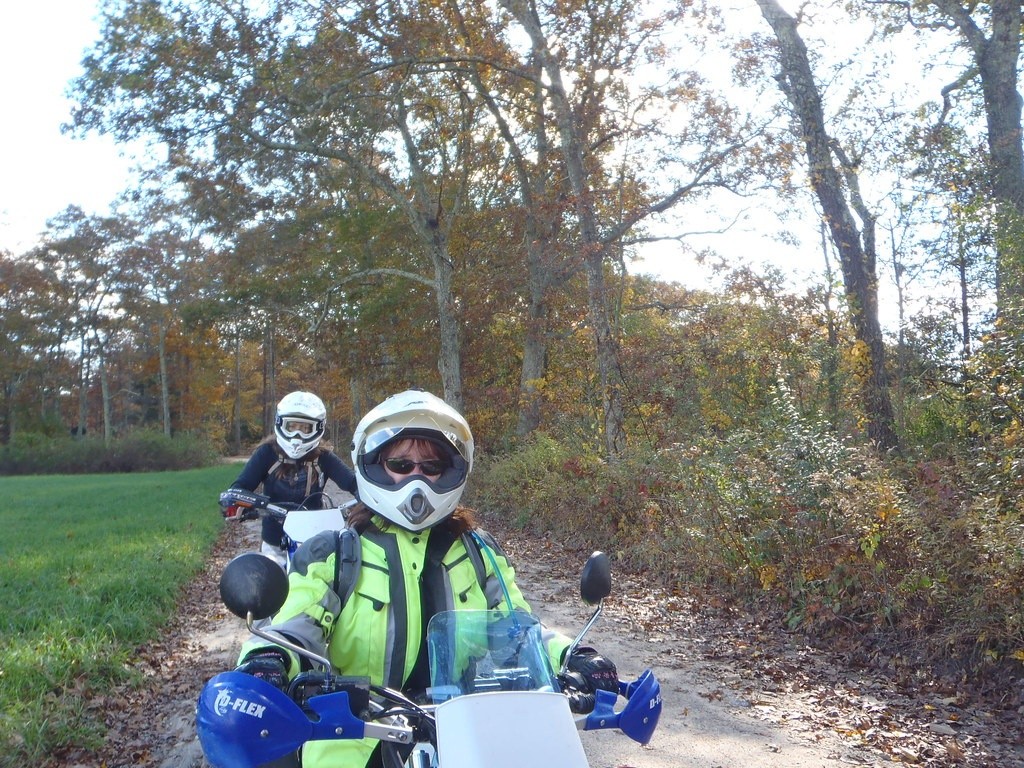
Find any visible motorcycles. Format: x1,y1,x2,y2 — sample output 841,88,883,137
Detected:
196,549,663,768
220,488,357,572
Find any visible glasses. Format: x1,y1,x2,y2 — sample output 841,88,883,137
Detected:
380,455,448,476
278,417,323,440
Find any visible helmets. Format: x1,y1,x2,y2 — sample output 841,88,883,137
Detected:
349,388,474,532
273,391,326,459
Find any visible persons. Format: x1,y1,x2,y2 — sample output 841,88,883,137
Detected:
217,391,361,553
235,389,620,768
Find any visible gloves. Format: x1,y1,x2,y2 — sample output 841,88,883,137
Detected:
240,652,289,694
227,506,239,518
567,646,619,696
